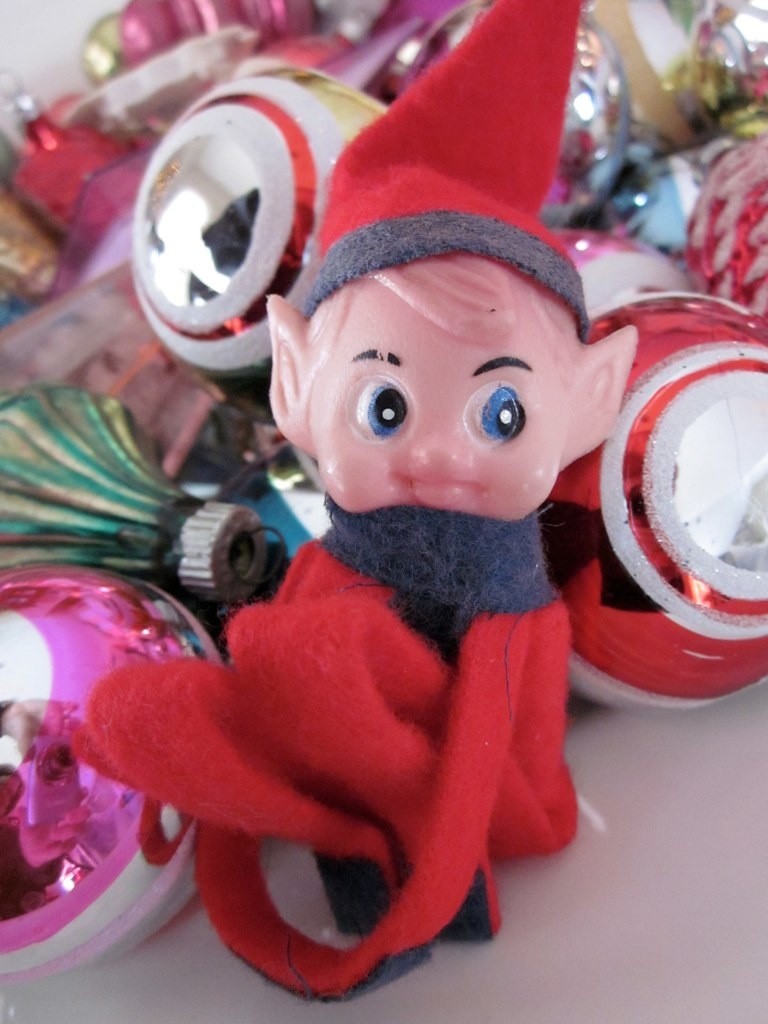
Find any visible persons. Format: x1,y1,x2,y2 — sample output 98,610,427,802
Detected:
72,0,641,1007
